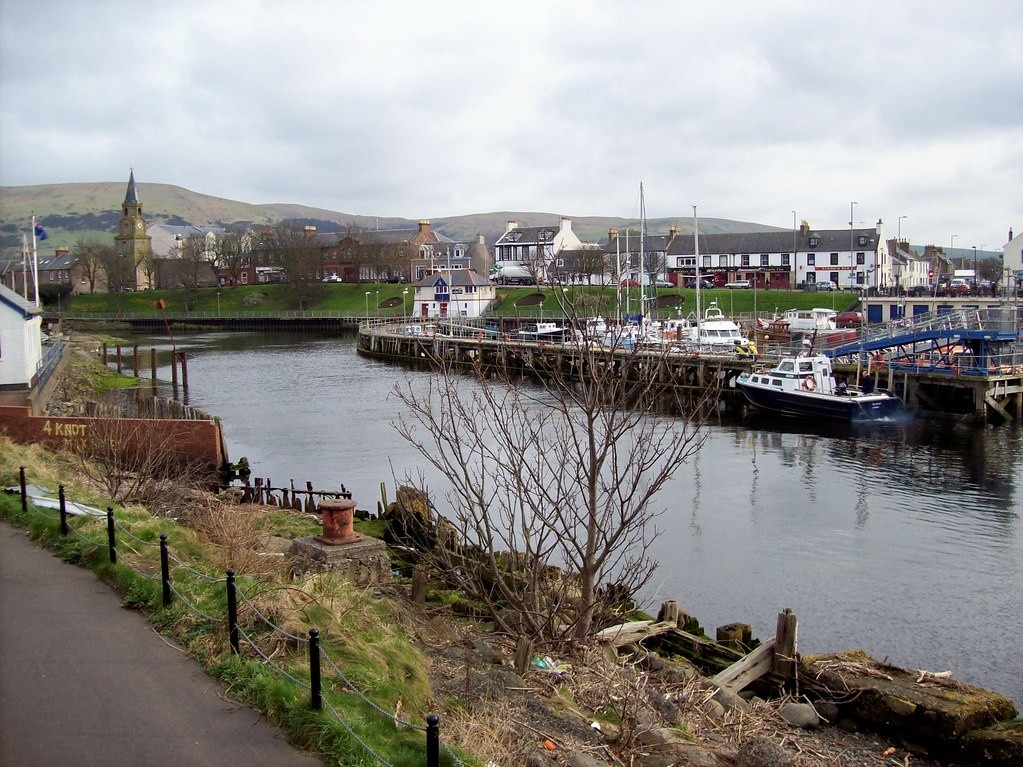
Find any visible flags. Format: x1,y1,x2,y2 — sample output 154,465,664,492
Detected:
34,222,48,241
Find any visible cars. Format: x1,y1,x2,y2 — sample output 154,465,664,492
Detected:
541,278,567,286
685,279,714,289
944,284,971,295
829,311,864,323
652,279,674,288
815,280,836,291
322,276,342,283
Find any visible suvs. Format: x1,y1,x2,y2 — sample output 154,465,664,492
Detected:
725,280,751,289
385,276,406,284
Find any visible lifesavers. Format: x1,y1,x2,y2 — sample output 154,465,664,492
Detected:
871,354,885,370
803,374,817,391
477,333,483,343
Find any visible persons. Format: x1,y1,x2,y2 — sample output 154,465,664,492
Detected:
765,278,770,290
861,370,874,394
946,279,950,287
991,281,996,298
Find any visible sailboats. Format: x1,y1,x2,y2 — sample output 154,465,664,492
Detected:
604,182,749,354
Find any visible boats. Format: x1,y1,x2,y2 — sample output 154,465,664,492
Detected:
570,316,607,341
916,344,974,368
512,322,569,341
735,357,903,423
404,324,448,338
749,307,857,349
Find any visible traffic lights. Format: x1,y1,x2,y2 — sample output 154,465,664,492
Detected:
929,270,933,277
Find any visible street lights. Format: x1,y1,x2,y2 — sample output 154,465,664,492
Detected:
660,237,666,281
365,292,370,329
792,210,797,289
972,245,976,286
851,202,858,292
951,234,957,261
403,291,409,323
898,216,907,276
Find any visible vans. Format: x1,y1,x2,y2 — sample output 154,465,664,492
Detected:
951,279,965,287
